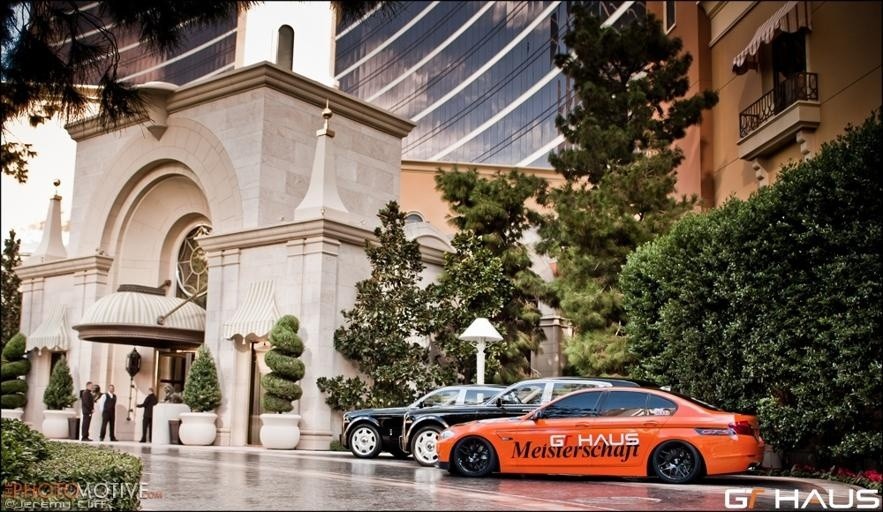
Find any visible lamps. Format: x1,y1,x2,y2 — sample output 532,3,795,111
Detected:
125,346,141,421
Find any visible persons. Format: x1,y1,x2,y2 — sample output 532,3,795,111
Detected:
98,385,118,441
79,380,96,442
134,386,157,443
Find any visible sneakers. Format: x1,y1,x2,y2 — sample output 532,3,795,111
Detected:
81,436,151,445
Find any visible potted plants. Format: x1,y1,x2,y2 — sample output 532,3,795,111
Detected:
0,332,31,421
259,314,304,449
42,357,76,438
179,344,222,447
